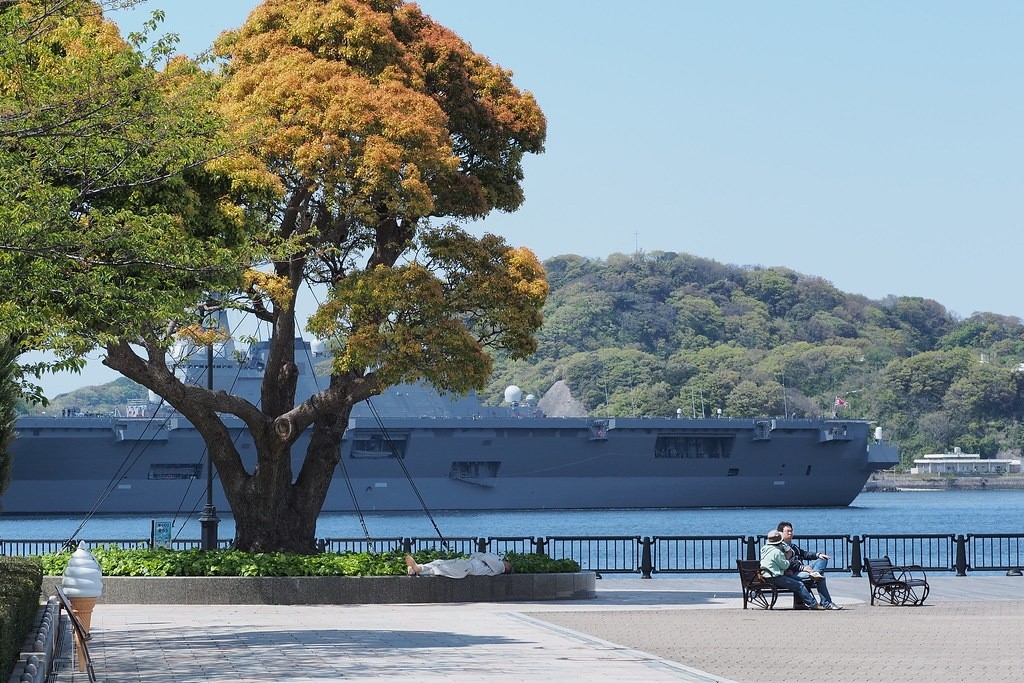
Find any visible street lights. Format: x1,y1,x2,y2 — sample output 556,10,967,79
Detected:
192,293,221,549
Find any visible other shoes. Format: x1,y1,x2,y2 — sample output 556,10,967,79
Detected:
811,604,825,609
794,602,811,609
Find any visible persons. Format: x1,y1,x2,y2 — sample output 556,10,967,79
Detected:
84,411,89,417
757,521,843,609
71,408,76,415
759,529,826,609
405,552,512,579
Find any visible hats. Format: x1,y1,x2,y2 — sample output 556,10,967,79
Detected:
766,530,784,544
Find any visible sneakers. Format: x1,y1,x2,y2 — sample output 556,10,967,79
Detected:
809,572,825,582
825,602,843,609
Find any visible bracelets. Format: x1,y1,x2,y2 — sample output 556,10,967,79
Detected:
757,572,759,574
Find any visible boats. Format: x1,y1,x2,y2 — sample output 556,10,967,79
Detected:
353,450,393,459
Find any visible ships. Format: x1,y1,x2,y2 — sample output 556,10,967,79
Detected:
0,274,899,515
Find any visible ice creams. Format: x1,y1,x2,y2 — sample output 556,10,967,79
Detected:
62,540,104,673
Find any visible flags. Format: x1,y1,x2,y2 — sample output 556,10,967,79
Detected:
836,398,848,409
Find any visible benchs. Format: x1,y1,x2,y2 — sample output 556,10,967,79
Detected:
863,555,930,606
735,558,820,610
